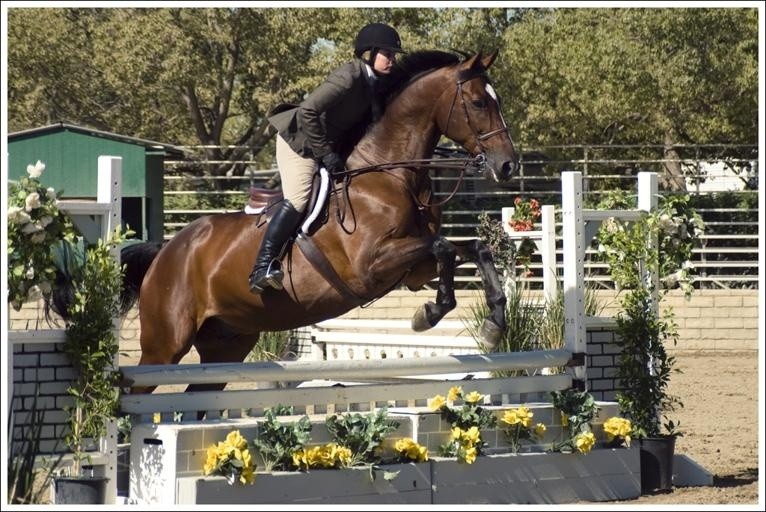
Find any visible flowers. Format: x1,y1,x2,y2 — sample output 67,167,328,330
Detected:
205,406,429,486
509,196,542,233
8,159,67,316
428,386,635,464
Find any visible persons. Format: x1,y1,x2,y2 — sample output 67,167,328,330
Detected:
248,23,407,295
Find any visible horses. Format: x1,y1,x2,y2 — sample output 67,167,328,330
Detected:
48,41,524,423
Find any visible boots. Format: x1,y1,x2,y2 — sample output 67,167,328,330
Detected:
247,199,305,294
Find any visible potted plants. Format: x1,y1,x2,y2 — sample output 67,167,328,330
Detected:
46,222,136,503
614,276,685,493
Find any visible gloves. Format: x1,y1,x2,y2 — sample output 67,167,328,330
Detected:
322,153,344,180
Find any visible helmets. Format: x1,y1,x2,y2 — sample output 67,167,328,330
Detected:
355,22,408,55
